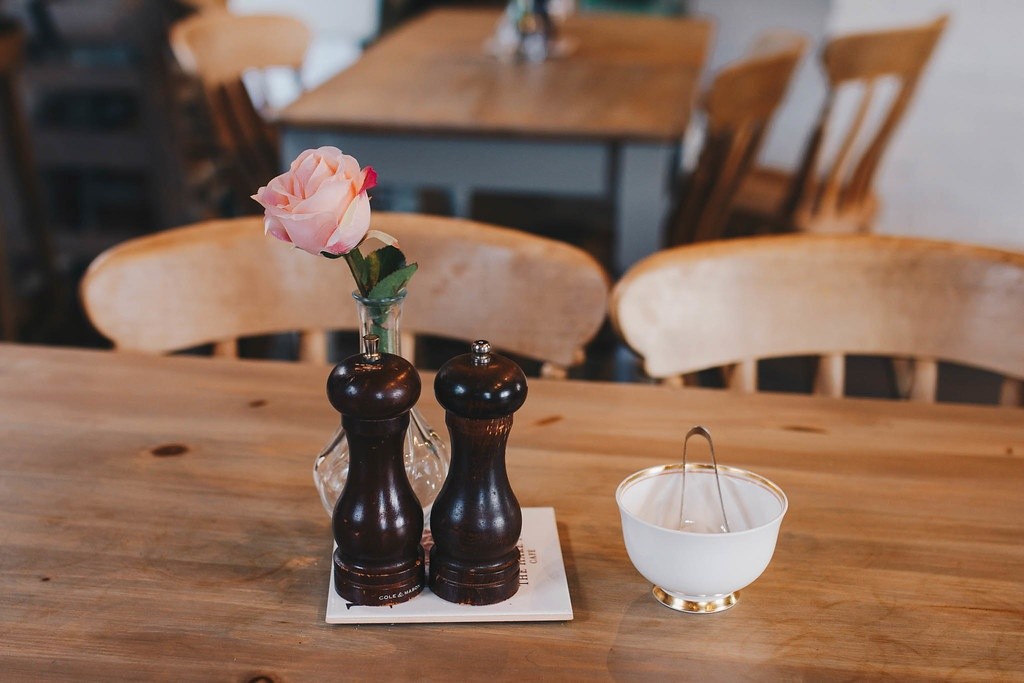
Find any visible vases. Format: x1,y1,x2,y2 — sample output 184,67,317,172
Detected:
312,287,448,531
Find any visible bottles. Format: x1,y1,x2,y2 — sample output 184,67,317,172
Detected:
327,334,427,605
428,338,529,606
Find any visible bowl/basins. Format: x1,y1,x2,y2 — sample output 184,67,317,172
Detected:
614,461,789,614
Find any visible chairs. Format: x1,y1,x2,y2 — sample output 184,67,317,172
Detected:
671,29,807,246
80,213,608,379
608,241,1023,406
168,12,312,214
681,15,948,233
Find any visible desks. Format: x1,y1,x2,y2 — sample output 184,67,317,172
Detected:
270,7,717,384
0,343,1023,682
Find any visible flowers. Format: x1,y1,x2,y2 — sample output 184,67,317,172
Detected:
249,147,418,300
